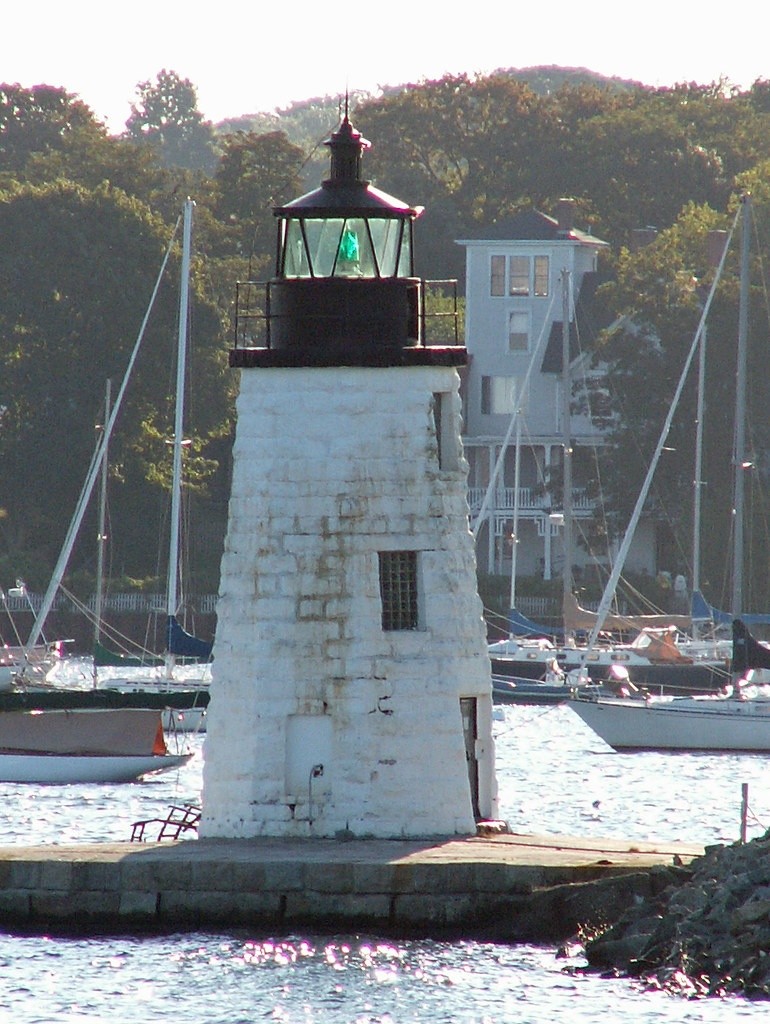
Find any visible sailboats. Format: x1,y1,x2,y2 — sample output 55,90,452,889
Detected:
0,198,215,785
470,191,770,754
